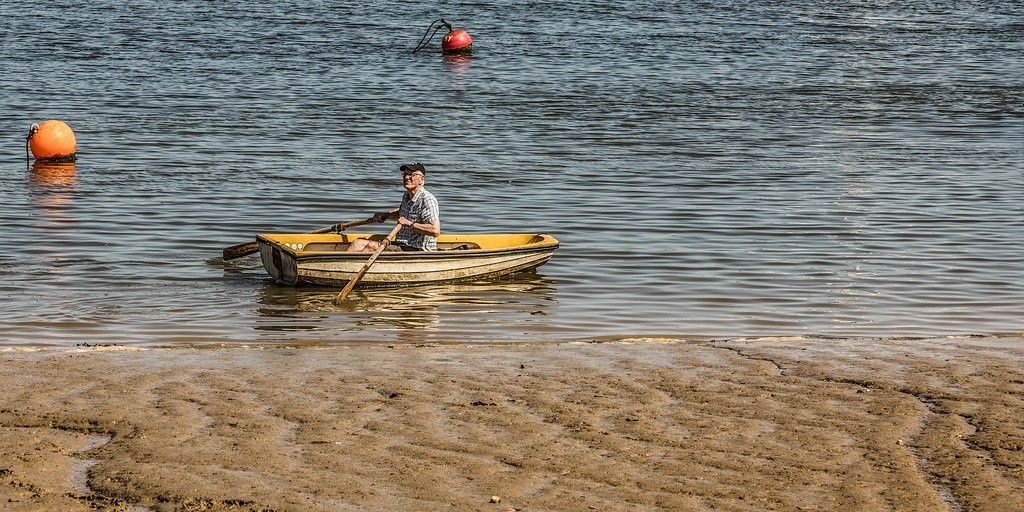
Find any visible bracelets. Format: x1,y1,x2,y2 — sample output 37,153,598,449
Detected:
409,221,415,228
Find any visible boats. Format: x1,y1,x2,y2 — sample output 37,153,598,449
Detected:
255,232,561,288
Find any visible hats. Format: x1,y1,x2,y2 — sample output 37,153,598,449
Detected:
400,163,425,175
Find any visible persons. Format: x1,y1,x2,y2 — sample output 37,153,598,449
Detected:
346,162,441,252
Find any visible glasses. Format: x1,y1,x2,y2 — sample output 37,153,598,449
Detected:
401,173,422,177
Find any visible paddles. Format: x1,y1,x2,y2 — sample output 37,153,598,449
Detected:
333,222,403,304
223,207,400,261
